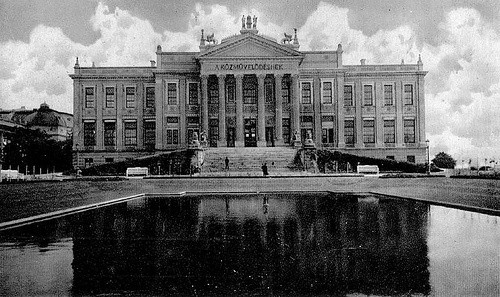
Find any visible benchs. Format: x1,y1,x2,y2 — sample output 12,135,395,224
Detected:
126,167,149,177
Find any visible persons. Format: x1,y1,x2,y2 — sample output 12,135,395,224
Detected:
246,14,252,28
253,15,258,28
225,196,230,212
262,163,268,175
263,195,270,215
241,14,247,29
225,157,230,168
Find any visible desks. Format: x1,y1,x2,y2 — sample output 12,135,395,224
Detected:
357,165,380,175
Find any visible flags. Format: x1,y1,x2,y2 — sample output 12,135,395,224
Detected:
462,156,500,165
